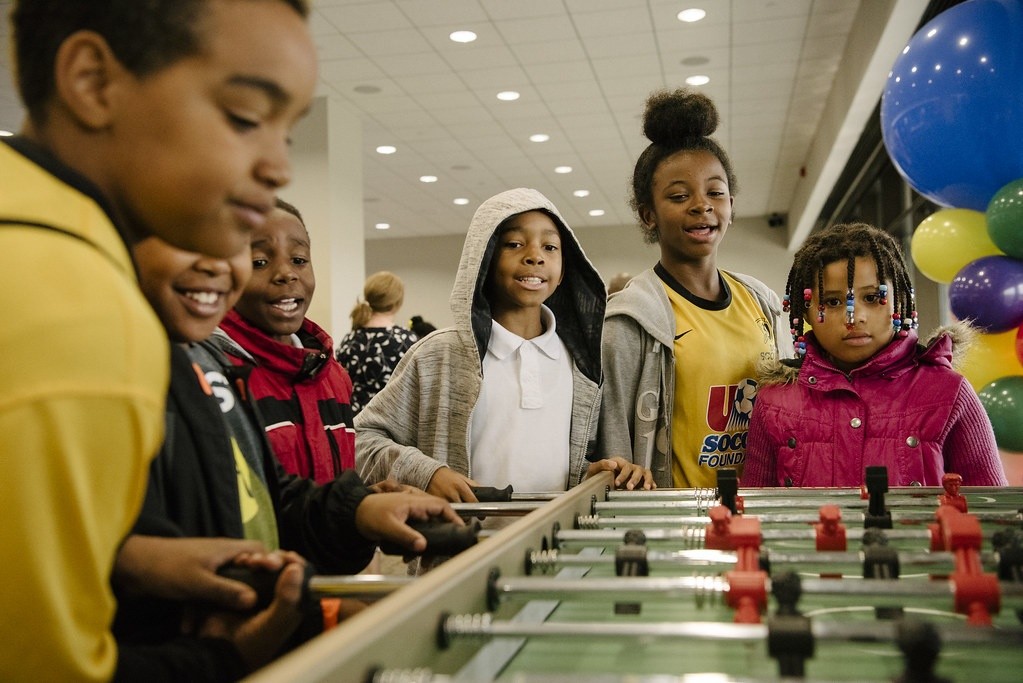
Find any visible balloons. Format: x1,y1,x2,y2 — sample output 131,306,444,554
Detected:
880,1,1023,488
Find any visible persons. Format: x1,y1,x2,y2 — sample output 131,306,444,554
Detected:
742,224,1009,486
0,0,657,683
599,91,796,489
